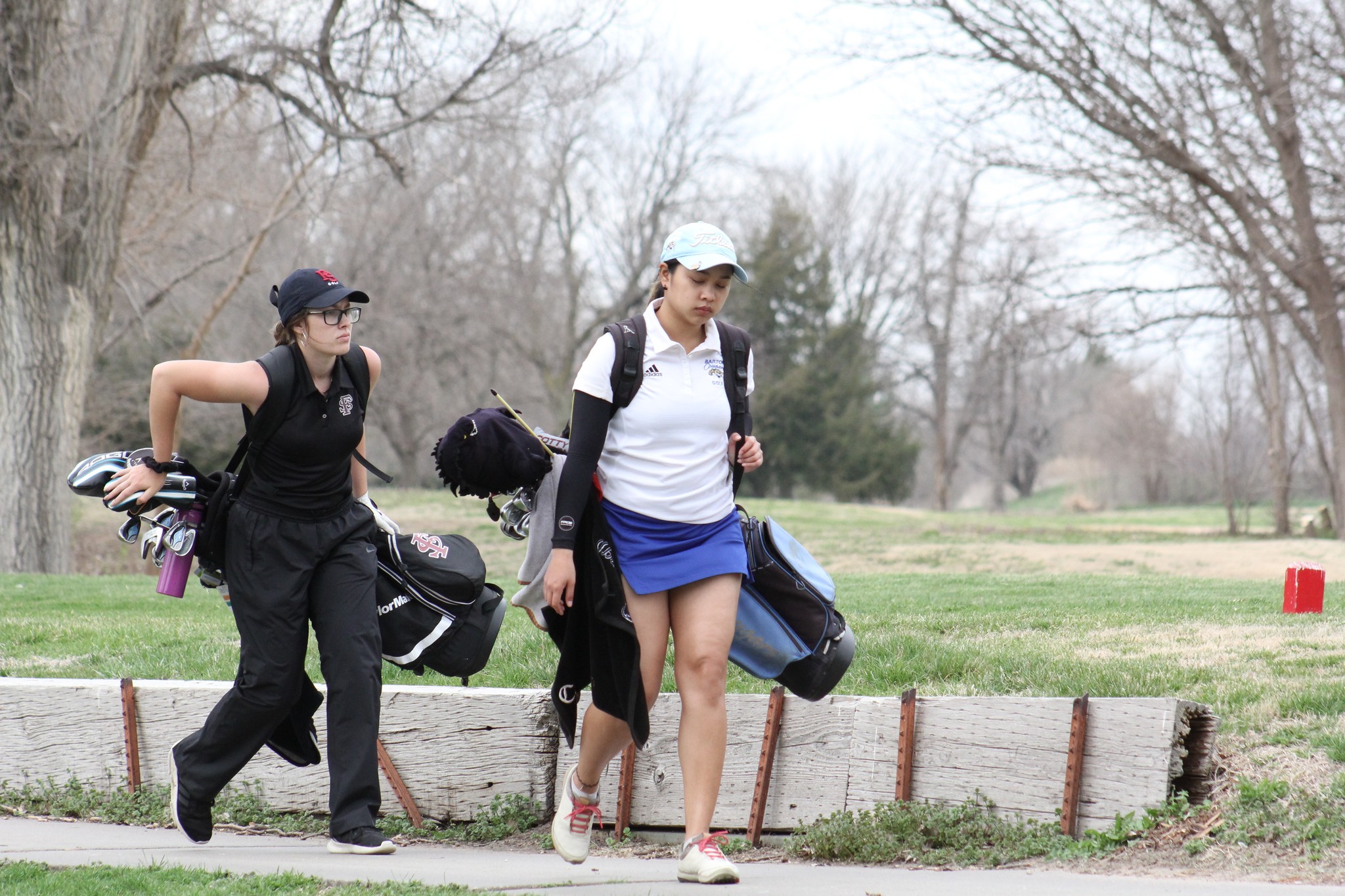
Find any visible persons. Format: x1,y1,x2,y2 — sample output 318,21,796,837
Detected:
103,269,396,854
543,221,763,883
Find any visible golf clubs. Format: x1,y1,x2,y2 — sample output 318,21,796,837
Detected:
116,507,198,570
499,486,535,540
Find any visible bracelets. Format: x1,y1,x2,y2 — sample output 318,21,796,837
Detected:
142,456,178,473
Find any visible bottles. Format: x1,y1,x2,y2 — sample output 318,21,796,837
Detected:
156,503,202,598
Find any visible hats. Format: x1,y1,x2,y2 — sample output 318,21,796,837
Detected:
660,222,751,285
271,269,370,326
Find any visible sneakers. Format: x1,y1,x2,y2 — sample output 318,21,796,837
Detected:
167,739,213,845
677,835,741,885
328,828,397,857
552,761,601,864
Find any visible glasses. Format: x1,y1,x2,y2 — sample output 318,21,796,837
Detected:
310,306,362,325
